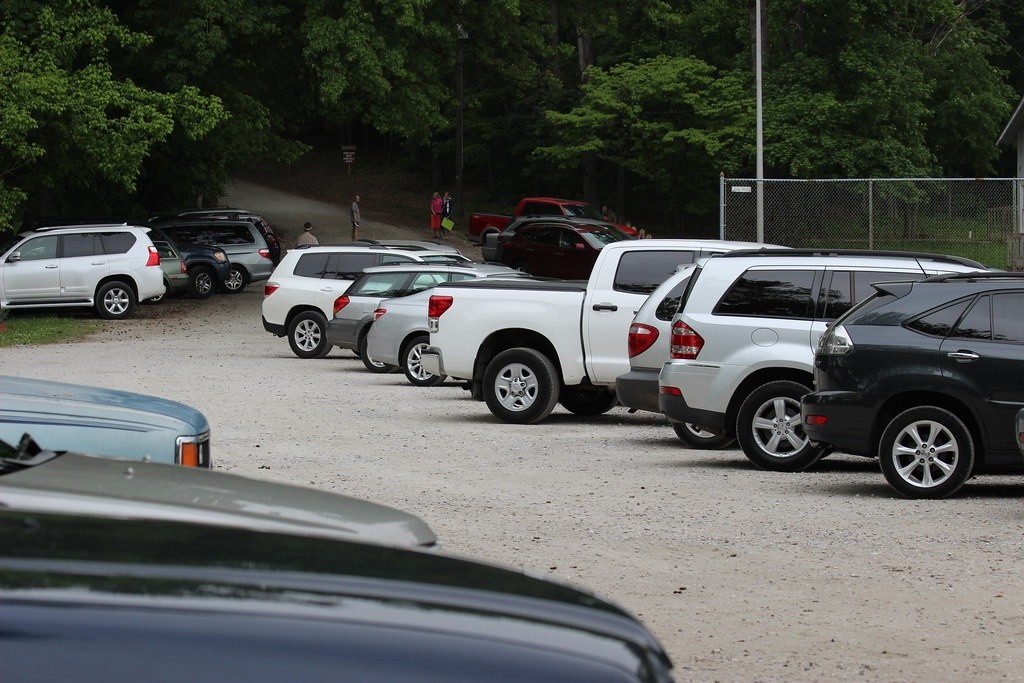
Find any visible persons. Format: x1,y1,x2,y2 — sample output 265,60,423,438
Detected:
351,194,360,241
441,191,453,241
430,192,444,238
290,222,320,249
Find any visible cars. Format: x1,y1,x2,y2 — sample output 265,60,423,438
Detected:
153,218,273,292
0,438,444,559
144,229,190,305
0,507,675,683
0,373,216,473
492,214,673,287
614,260,738,450
365,287,445,387
357,237,462,255
171,238,233,298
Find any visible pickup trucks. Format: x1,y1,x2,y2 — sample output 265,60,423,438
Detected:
417,238,793,427
466,195,642,250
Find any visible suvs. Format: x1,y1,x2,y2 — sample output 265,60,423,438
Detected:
260,241,472,359
0,223,166,319
148,207,281,264
655,247,1005,473
800,269,1024,499
324,259,525,374
